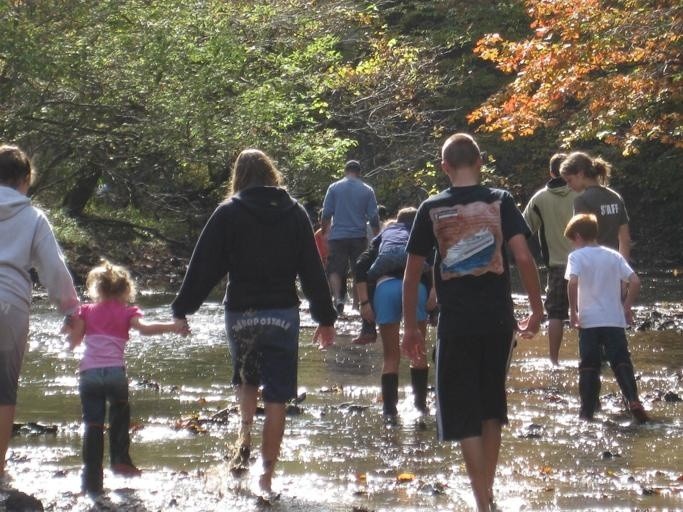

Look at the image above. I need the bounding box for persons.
[0,145,83,512]
[61,260,190,493]
[400,131,544,512]
[522,151,586,368]
[311,159,435,419]
[170,146,340,496]
[561,213,645,423]
[559,151,629,300]
